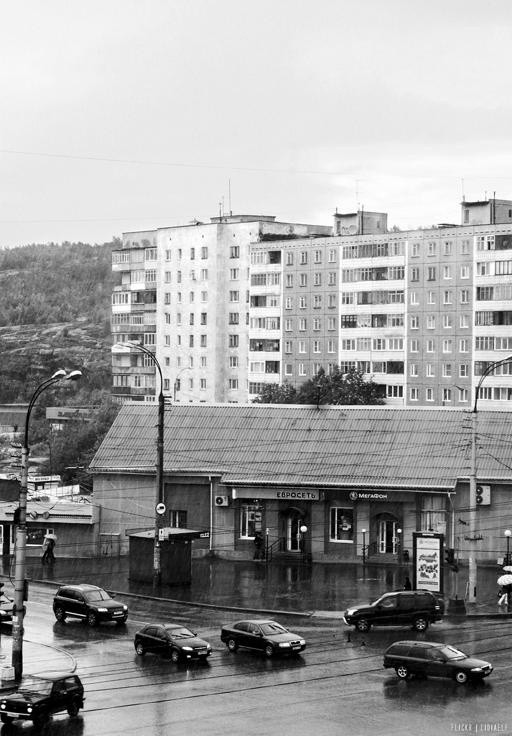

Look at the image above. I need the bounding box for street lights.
[10,367,85,680]
[115,340,165,598]
[361,528,367,578]
[503,529,512,566]
[300,525,308,579]
[396,528,403,564]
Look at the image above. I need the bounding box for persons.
[253,534,266,561]
[404,577,411,591]
[41,538,56,565]
[403,552,409,566]
[497,585,508,606]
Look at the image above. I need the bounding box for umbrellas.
[43,534,57,540]
[497,574,512,585]
[503,566,512,572]
[402,550,408,552]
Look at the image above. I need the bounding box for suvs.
[51,583,129,628]
[342,591,445,633]
[0,670,85,729]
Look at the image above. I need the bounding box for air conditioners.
[474,485,491,505]
[214,495,229,507]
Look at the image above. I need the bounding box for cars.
[133,622,214,664]
[382,640,494,684]
[219,619,307,657]
